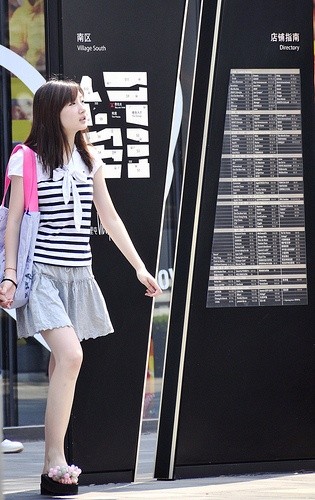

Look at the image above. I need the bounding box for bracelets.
[1,279,18,288]
[2,267,17,272]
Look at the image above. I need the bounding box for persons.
[1,76,160,495]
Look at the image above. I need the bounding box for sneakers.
[1,438,23,452]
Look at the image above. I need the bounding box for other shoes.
[40,465,82,495]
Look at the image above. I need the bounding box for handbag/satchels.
[0,143,40,308]
